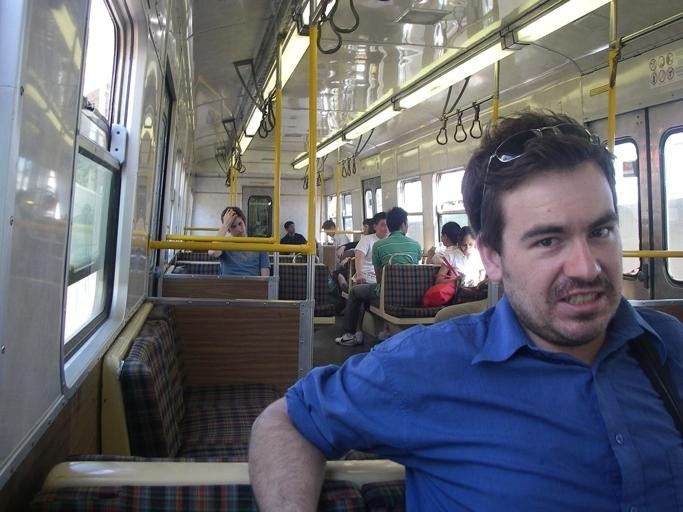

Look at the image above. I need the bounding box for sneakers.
[335,331,364,346]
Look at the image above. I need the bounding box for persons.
[352,212,392,346]
[279,220,308,244]
[426,221,463,266]
[322,221,351,247]
[208,207,272,278]
[328,218,378,312]
[334,207,422,347]
[436,226,487,304]
[248,110,683,509]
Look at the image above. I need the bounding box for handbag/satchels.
[423,277,458,306]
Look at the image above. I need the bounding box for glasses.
[479,124,596,230]
